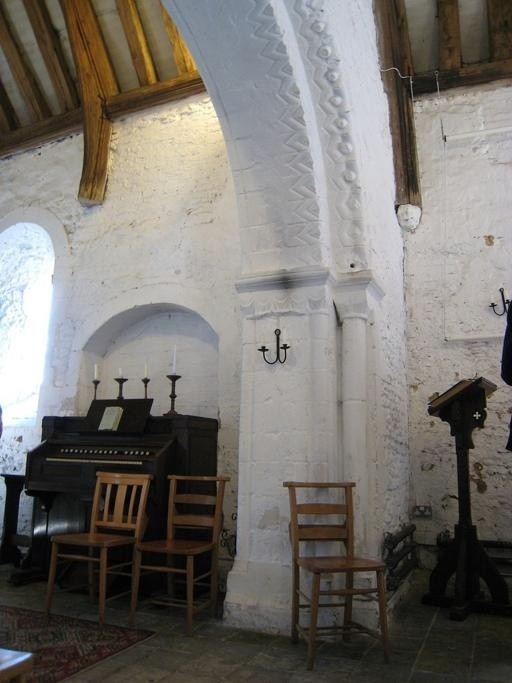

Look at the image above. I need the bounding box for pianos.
[7,398,216,597]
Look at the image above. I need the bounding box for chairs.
[44,472,390,671]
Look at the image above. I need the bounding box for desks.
[0,645,33,681]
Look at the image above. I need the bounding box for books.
[97,405,125,433]
[425,376,474,409]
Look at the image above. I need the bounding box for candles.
[94,344,178,381]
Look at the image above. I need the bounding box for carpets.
[0,604,156,683]
